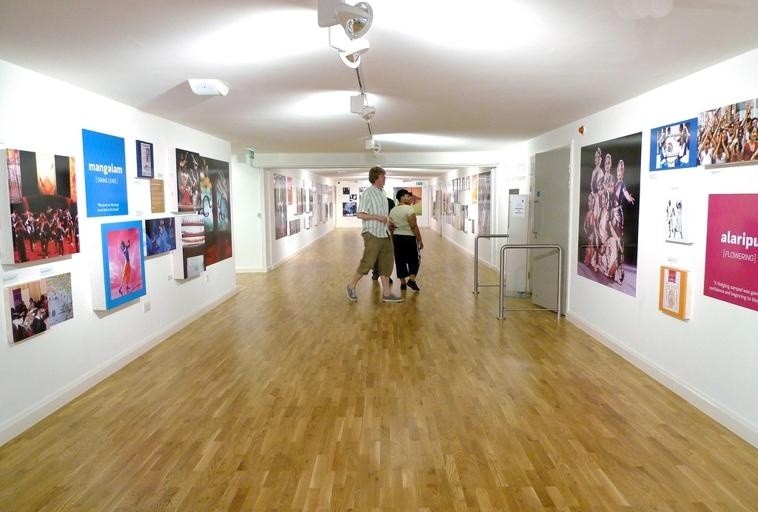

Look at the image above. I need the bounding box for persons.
[389,188,424,291]
[371,197,395,283]
[346,166,404,304]
[655,103,758,171]
[119,239,132,292]
[582,147,635,283]
[11,295,48,334]
[10,205,79,262]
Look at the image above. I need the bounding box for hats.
[396,189,412,200]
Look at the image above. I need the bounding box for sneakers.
[346,283,359,302]
[399,279,420,292]
[380,293,404,303]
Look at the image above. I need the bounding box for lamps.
[315,0,381,156]
